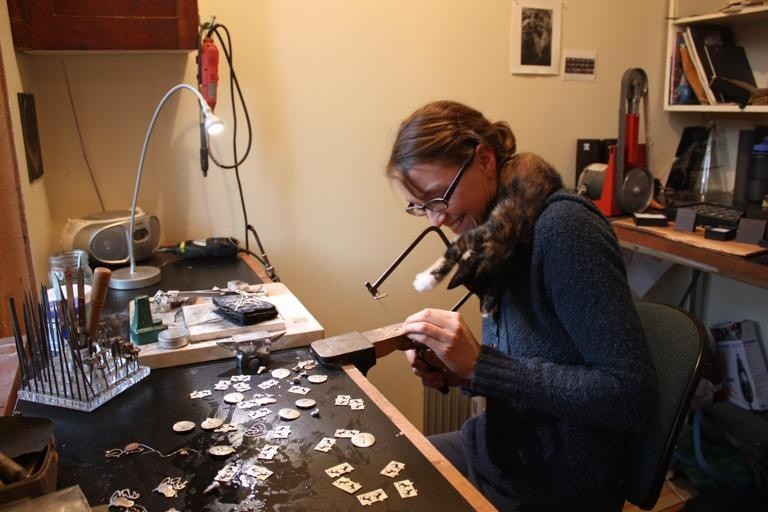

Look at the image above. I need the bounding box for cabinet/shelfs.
[6,0,200,57]
[663,0,768,114]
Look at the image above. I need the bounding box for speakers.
[576,140,602,192]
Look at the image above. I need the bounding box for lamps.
[108,84,226,290]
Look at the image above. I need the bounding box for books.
[667,25,758,105]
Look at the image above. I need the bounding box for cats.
[412,152,561,319]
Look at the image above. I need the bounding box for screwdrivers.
[86,268,112,385]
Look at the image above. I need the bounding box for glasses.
[405,154,476,216]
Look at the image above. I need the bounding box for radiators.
[421,385,473,436]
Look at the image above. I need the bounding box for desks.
[0,237,500,512]
[604,211,768,322]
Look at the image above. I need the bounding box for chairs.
[626,302,709,510]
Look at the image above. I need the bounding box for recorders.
[60,207,162,271]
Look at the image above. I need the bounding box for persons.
[383,99,658,511]
